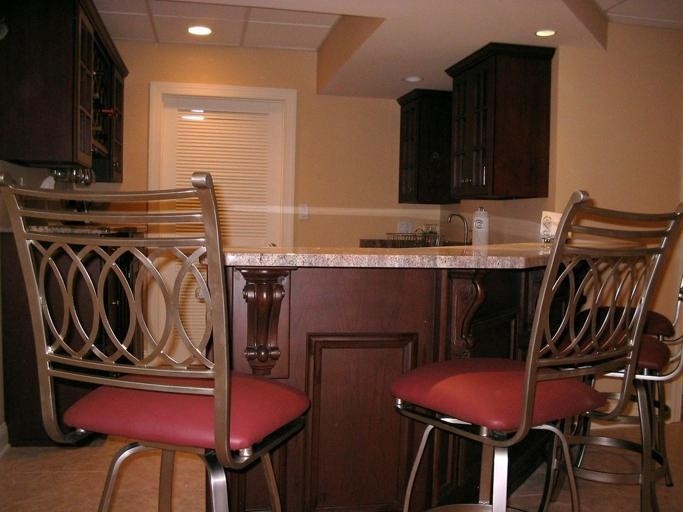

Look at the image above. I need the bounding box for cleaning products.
[473,207,489,246]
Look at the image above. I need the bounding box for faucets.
[448,213,468,245]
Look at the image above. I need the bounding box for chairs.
[1,172,311,512]
[550,276,683,512]
[392,188,683,511]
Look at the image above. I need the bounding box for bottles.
[471,206,490,246]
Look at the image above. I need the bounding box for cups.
[424,224,437,247]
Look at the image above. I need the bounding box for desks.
[147,242,649,512]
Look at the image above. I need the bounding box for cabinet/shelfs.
[444,41,556,200]
[398,88,464,204]
[0,1,130,447]
